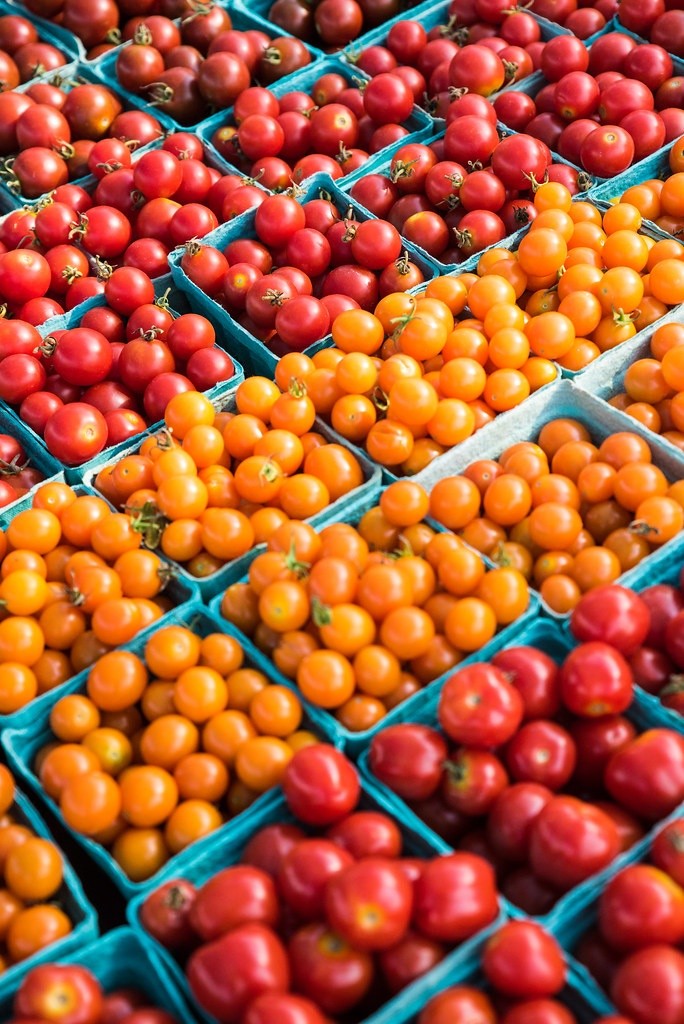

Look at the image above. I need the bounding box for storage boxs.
[0,0,684,1024]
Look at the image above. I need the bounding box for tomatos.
[0,0,684,1024]
